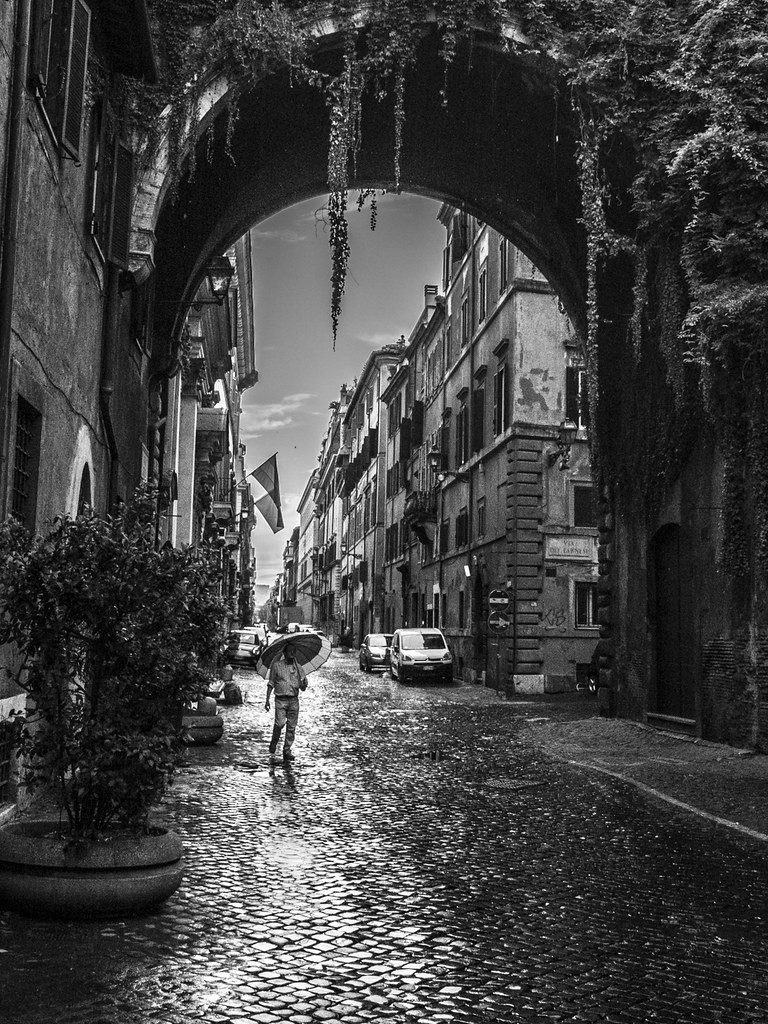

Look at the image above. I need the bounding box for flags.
[252,454,281,509]
[255,494,284,534]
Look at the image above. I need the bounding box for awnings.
[284,560,293,570]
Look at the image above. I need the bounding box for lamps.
[426,443,475,483]
[545,416,578,471]
[340,538,363,560]
[300,587,308,597]
[154,241,234,306]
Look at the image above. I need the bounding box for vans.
[386,627,455,685]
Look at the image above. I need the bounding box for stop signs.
[487,589,510,611]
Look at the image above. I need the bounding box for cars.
[285,622,325,638]
[359,633,395,672]
[243,622,273,651]
[217,629,265,671]
[587,642,609,694]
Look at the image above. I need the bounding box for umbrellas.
[256,631,332,679]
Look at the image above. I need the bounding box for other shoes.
[283,750,295,759]
[269,742,276,753]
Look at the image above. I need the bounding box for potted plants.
[0,473,233,915]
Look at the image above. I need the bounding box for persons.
[265,645,308,761]
[281,624,288,631]
